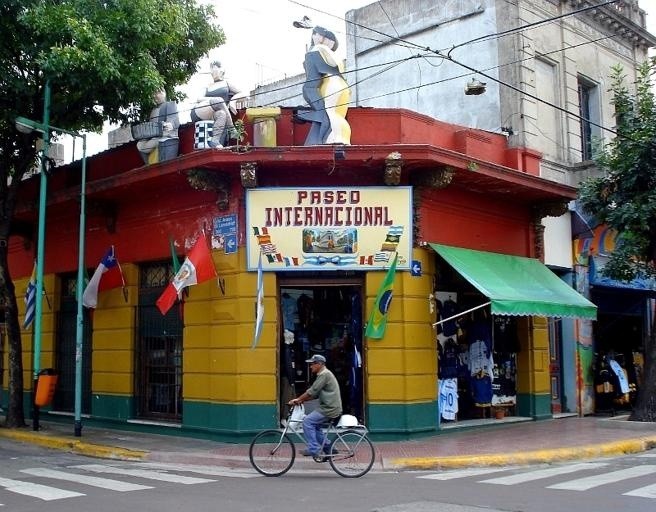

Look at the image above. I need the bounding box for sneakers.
[301,447,340,462]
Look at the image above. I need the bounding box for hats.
[304,353,327,363]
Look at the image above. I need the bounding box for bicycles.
[248,398,375,479]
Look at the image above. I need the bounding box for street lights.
[14,114,88,436]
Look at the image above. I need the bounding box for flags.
[154,231,219,317]
[249,252,270,347]
[363,253,399,340]
[20,259,46,332]
[79,245,127,310]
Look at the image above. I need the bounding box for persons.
[135,86,184,168]
[306,30,355,145]
[293,27,343,143]
[287,353,343,459]
[187,61,248,150]
